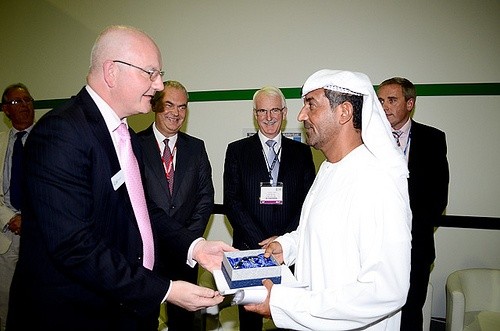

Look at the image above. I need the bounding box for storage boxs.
[221,248,281,289]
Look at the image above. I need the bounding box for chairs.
[446,268,500,331]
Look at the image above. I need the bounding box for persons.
[223,86,316,331]
[136,81,216,331]
[0,83,37,331]
[377,77,449,331]
[244,69,413,331]
[6,25,241,331]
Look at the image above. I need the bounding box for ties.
[116,124,155,271]
[161,139,175,196]
[392,132,403,146]
[266,140,280,187]
[9,131,27,212]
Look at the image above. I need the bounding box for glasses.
[254,107,285,115]
[6,97,35,105]
[113,60,166,82]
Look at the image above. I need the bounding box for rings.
[15,231,18,233]
